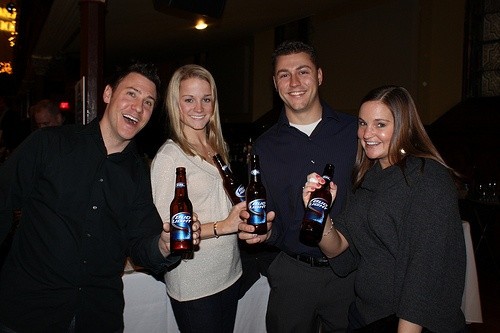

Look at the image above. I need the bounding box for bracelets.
[213,220,220,238]
[322,218,334,237]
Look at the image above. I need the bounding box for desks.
[468,197,500,265]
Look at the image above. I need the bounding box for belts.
[282,250,330,268]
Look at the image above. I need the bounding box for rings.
[302,186,305,191]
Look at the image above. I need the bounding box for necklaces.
[197,146,211,158]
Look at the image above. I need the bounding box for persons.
[0,63,201,333]
[238,40,359,333]
[302,83,467,333]
[29,98,63,128]
[149,64,247,333]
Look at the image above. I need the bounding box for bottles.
[247,136,253,154]
[298,163,335,247]
[213,153,246,205]
[170,167,194,259]
[247,153,267,235]
